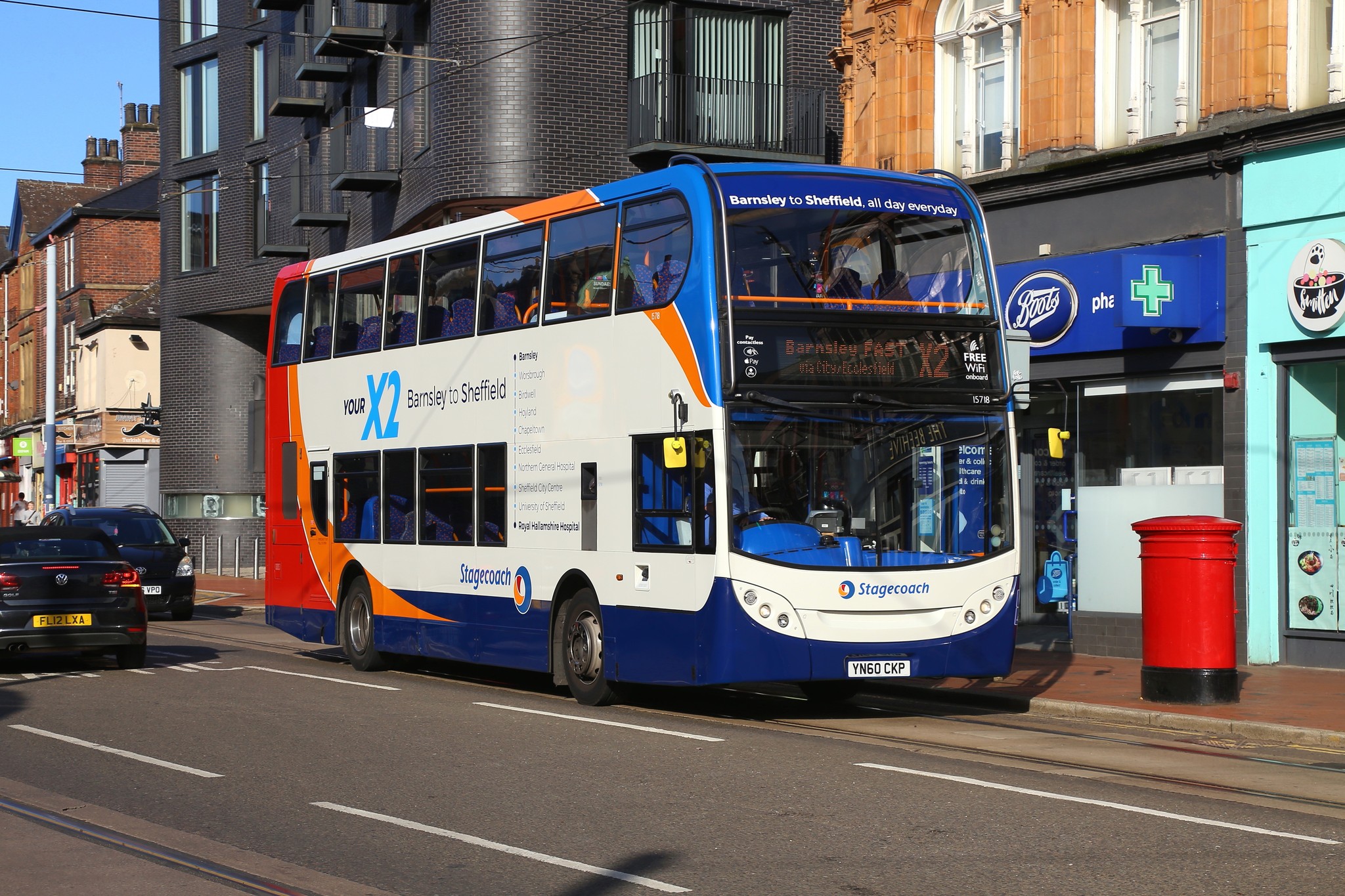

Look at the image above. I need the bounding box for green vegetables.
[1303,596,1322,613]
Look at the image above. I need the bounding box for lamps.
[84,338,98,352]
[128,334,144,343]
[67,344,84,352]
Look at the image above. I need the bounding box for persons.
[699,467,776,547]
[10,492,29,527]
[21,501,41,526]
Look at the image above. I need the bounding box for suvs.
[38,504,195,622]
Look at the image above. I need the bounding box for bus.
[264,153,1071,707]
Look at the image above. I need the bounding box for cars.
[0,524,147,664]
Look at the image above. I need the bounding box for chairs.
[651,258,686,305]
[357,315,382,351]
[463,519,504,543]
[632,263,655,307]
[870,268,924,313]
[373,493,414,539]
[310,325,333,357]
[720,257,755,306]
[115,524,148,543]
[446,297,476,336]
[425,304,454,340]
[399,509,446,541]
[339,319,365,354]
[339,496,360,539]
[489,291,522,330]
[423,521,459,542]
[278,343,301,363]
[387,309,416,346]
[824,266,873,312]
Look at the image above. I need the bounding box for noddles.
[1299,604,1318,615]
